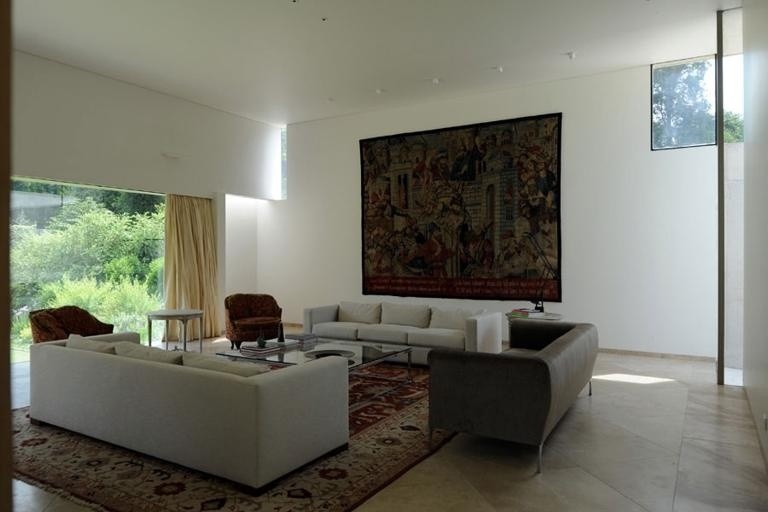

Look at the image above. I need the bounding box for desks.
[148,309,203,354]
[506,310,562,321]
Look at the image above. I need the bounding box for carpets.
[12,361,454,512]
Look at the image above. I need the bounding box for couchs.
[29,305,115,343]
[303,300,477,366]
[427,318,600,474]
[28,332,350,496]
[224,293,283,349]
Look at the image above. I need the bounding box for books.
[505,307,545,319]
[240,345,281,354]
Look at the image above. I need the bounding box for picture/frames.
[359,113,565,304]
